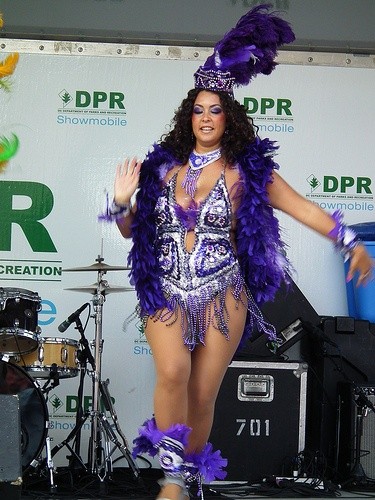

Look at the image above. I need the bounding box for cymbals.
[63,283,137,293]
[56,263,133,271]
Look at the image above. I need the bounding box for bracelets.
[328,209,361,263]
[96,188,132,222]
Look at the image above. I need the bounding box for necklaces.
[182,147,222,199]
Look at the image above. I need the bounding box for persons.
[97,4,375,500]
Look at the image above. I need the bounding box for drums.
[0,287,42,356]
[8,336,80,379]
[0,358,50,485]
